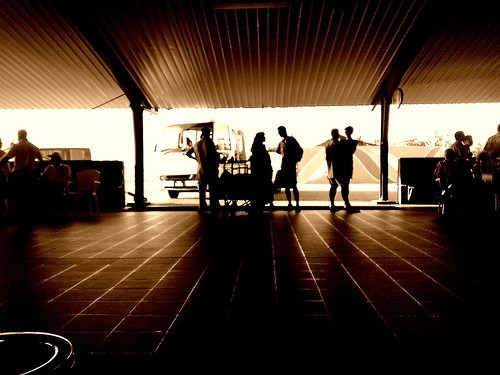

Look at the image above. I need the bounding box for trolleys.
[217,156,276,217]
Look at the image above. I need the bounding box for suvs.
[158,120,249,199]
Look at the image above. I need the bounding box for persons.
[187,127,219,210]
[249,132,277,217]
[437,122,500,229]
[0,128,72,228]
[324,128,361,214]
[270,126,302,212]
[339,126,356,200]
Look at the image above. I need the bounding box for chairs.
[76,169,100,217]
